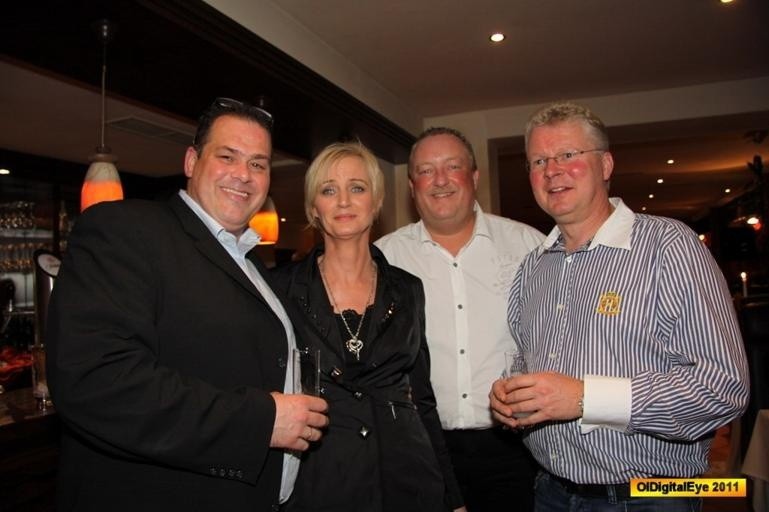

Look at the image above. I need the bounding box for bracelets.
[578,395,585,411]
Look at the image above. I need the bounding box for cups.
[292,345,321,398]
[30,345,50,400]
[503,349,536,430]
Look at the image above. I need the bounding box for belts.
[547,473,642,502]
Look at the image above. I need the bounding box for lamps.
[78,16,129,212]
[246,94,279,247]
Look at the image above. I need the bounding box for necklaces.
[319,259,377,361]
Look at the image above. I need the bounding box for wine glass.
[0,197,73,276]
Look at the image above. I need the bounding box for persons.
[267,138,469,511]
[486,100,753,510]
[374,126,549,512]
[45,99,331,511]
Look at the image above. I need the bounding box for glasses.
[210,96,277,131]
[525,147,608,174]
